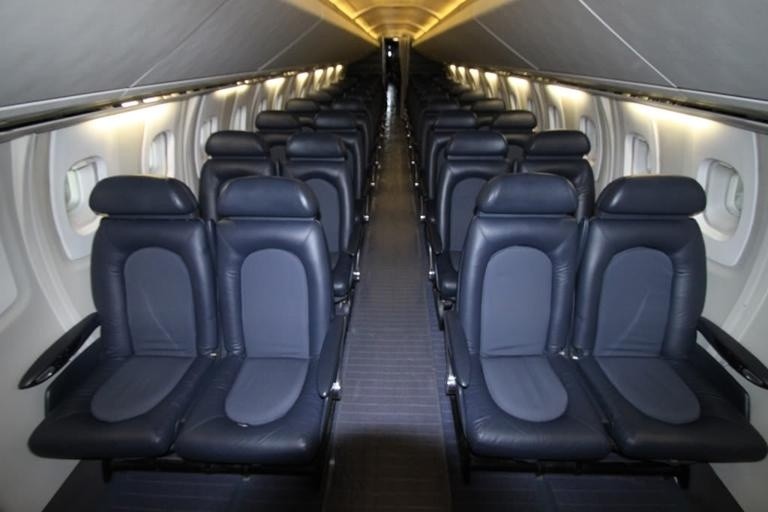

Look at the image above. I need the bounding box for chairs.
[426,129,523,296]
[282,136,362,304]
[470,97,509,116]
[171,174,344,476]
[18,174,208,475]
[313,110,362,138]
[335,98,367,112]
[442,169,613,476]
[421,96,468,116]
[286,97,319,115]
[195,130,278,218]
[419,115,476,134]
[514,130,597,216]
[487,108,539,136]
[253,109,304,152]
[578,172,764,477]
